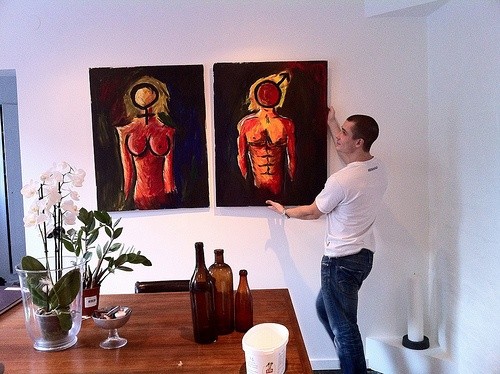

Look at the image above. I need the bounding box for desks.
[0,287,314,374]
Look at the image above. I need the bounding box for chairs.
[134,280,191,293]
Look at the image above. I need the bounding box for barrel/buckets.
[241,322,289,374]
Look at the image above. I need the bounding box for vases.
[14,255,87,351]
[34,304,72,342]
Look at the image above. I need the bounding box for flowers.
[21,159,87,331]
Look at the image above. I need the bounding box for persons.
[266,106,388,374]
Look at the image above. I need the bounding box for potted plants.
[46,206,152,317]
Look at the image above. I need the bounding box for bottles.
[234,269,254,333]
[208,249,234,336]
[189,241,218,344]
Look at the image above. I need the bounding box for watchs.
[283,208,290,219]
